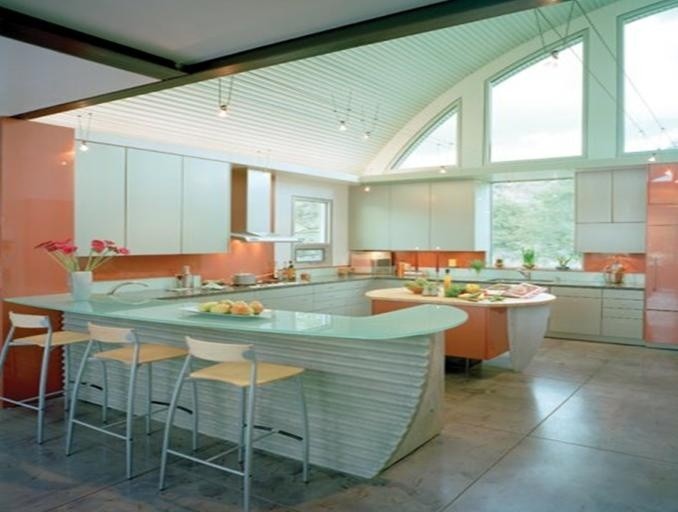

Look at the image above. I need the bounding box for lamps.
[217,76,234,116]
[77,114,92,151]
[331,94,351,132]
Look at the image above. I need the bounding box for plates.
[232,272,256,286]
[179,305,272,320]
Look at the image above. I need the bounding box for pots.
[602,261,626,286]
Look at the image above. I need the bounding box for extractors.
[229,233,299,244]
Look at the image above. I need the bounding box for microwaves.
[350,252,392,275]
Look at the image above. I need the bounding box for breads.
[249,300,262,311]
[203,300,216,311]
[229,304,252,317]
[219,297,235,309]
[210,303,229,313]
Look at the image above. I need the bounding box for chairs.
[0,311,108,446]
[157,336,310,512]
[64,322,197,481]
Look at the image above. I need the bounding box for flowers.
[32,237,129,271]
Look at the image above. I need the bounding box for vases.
[69,271,93,301]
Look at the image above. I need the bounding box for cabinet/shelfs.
[347,185,390,251]
[126,146,182,258]
[574,167,645,255]
[74,137,126,257]
[431,180,491,252]
[182,156,232,256]
[548,286,601,337]
[601,288,644,340]
[643,163,678,347]
[389,183,431,252]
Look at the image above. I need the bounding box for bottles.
[443,269,451,292]
